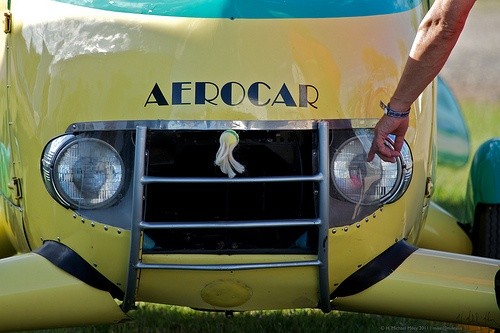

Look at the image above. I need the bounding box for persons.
[366,0,477,163]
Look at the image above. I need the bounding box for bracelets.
[379,100,411,118]
